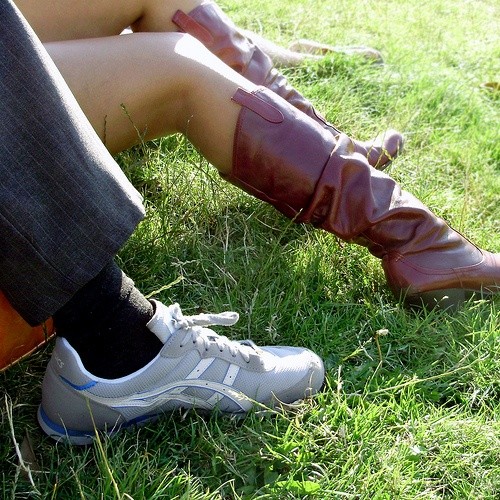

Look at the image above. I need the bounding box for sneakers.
[37,299,325,443]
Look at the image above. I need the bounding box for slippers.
[289,40,384,74]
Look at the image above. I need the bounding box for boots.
[218,86,500,309]
[173,1,404,178]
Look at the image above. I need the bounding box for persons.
[0,0,500,311]
[2,0,327,446]
